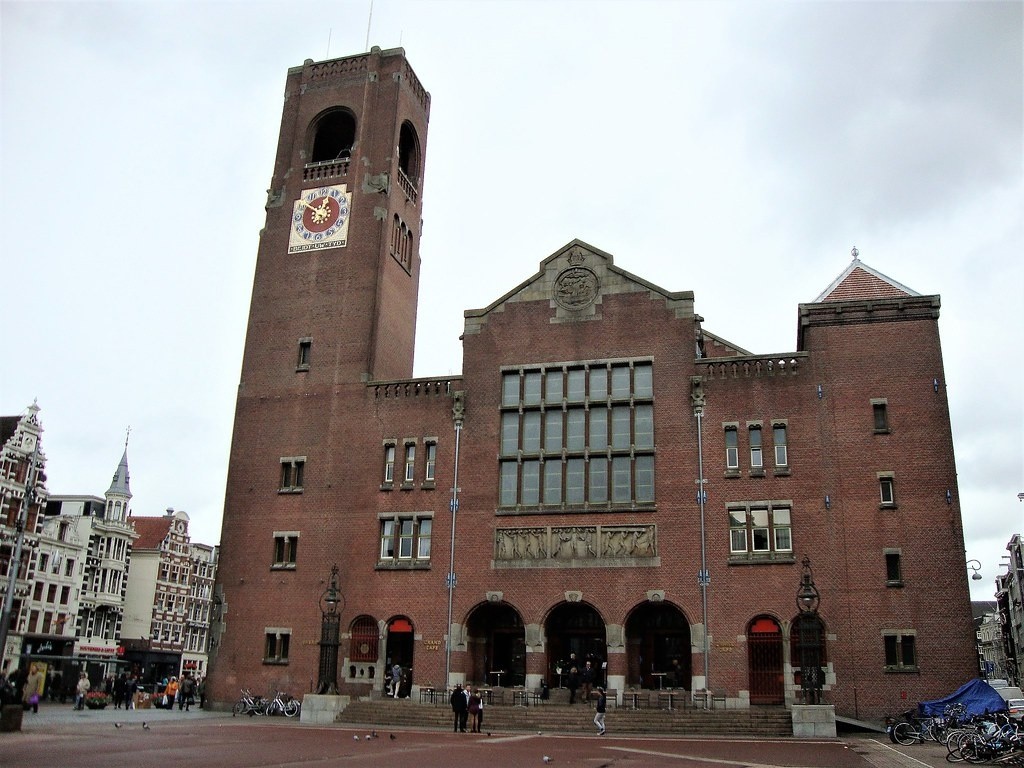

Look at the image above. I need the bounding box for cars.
[1006,699,1024,721]
[995,687,1023,701]
[983,680,1008,687]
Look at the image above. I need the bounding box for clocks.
[288,182,353,254]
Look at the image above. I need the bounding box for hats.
[597,687,604,691]
[171,676,175,679]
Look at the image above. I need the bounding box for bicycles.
[232,686,301,718]
[885,701,1024,767]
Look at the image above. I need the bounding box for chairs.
[529,688,544,707]
[637,694,650,709]
[674,694,687,710]
[589,691,600,708]
[493,691,505,705]
[606,689,618,709]
[713,694,726,710]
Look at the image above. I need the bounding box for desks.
[478,688,493,704]
[448,687,464,702]
[662,692,679,709]
[696,691,714,711]
[626,691,641,708]
[513,690,529,705]
[420,687,436,703]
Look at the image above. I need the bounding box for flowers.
[152,692,164,705]
[85,691,113,706]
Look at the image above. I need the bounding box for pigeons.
[114,722,124,729]
[372,729,380,739]
[364,734,372,740]
[389,733,398,741]
[352,733,360,741]
[141,720,151,731]
[537,730,542,735]
[486,732,491,737]
[541,753,555,765]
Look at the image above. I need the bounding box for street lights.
[791,555,826,704]
[312,563,341,695]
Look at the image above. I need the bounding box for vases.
[88,702,107,709]
[154,702,166,708]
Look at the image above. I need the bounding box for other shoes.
[394,696,399,699]
[388,693,393,695]
[597,730,605,735]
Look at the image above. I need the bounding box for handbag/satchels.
[163,696,168,705]
[188,697,195,705]
[130,702,135,709]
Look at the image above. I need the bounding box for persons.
[451,683,484,733]
[385,663,412,700]
[0,665,67,713]
[105,672,207,710]
[72,671,91,711]
[556,650,593,705]
[593,685,606,735]
